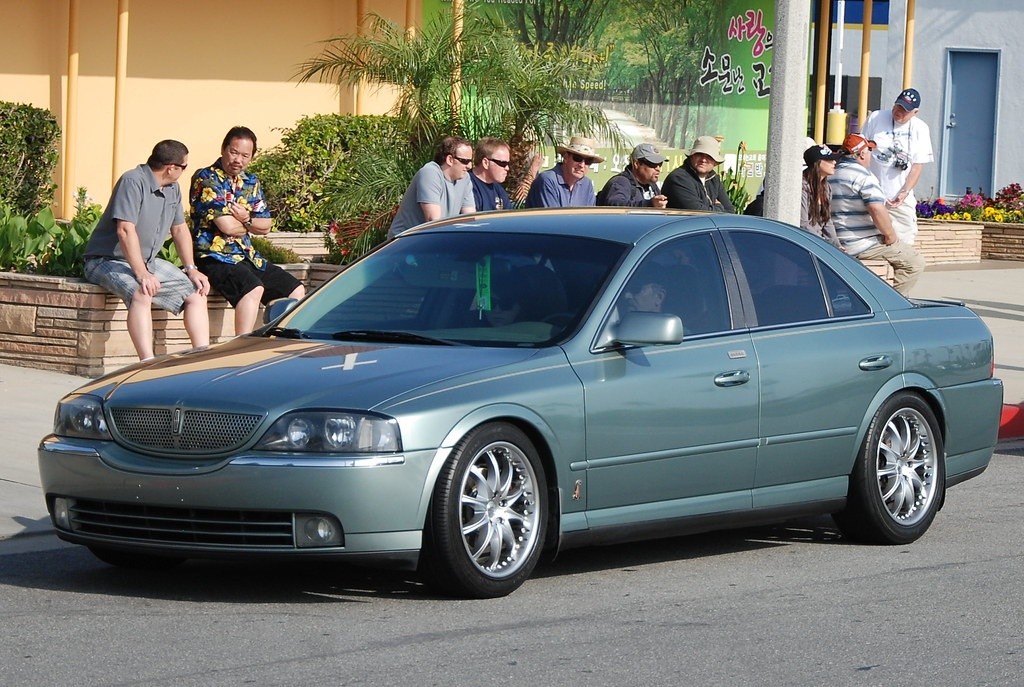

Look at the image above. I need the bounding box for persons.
[596,143,668,208]
[467,136,515,214]
[860,88,934,246]
[621,262,694,338]
[524,136,608,208]
[387,136,479,329]
[189,126,305,337]
[661,136,736,214]
[82,140,210,364]
[464,276,542,329]
[826,132,924,301]
[743,132,847,252]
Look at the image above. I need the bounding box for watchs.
[243,216,252,228]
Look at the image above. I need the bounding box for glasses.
[490,296,519,310]
[488,157,510,167]
[865,147,873,152]
[626,284,655,295]
[568,152,593,165]
[895,158,908,169]
[454,155,473,165]
[163,162,187,169]
[639,160,663,168]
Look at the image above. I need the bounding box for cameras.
[894,158,907,170]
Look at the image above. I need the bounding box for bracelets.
[900,189,909,195]
[184,265,198,272]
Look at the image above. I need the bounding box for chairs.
[458,258,541,325]
[669,264,711,334]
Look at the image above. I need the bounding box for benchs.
[754,285,828,327]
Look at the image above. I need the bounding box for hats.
[843,133,877,153]
[804,143,842,164]
[555,136,604,163]
[684,135,724,166]
[631,142,670,162]
[895,88,920,111]
[626,261,685,309]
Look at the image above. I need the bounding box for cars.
[38,207,1006,600]
